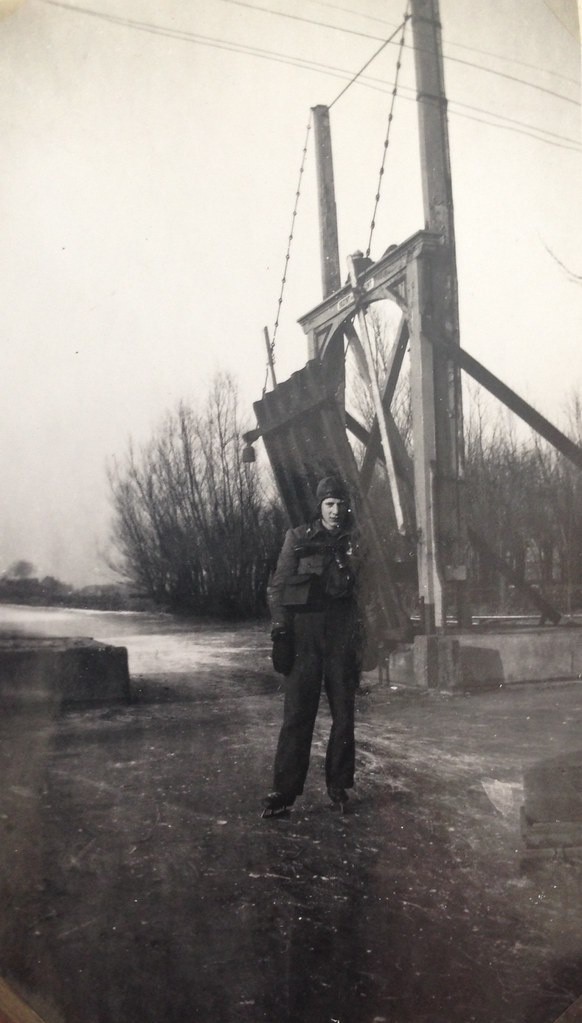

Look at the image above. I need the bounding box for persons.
[261,477,367,810]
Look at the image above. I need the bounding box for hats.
[314,478,350,508]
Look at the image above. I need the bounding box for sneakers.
[261,791,295,818]
[328,786,349,814]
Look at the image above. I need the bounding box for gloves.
[272,629,296,676]
